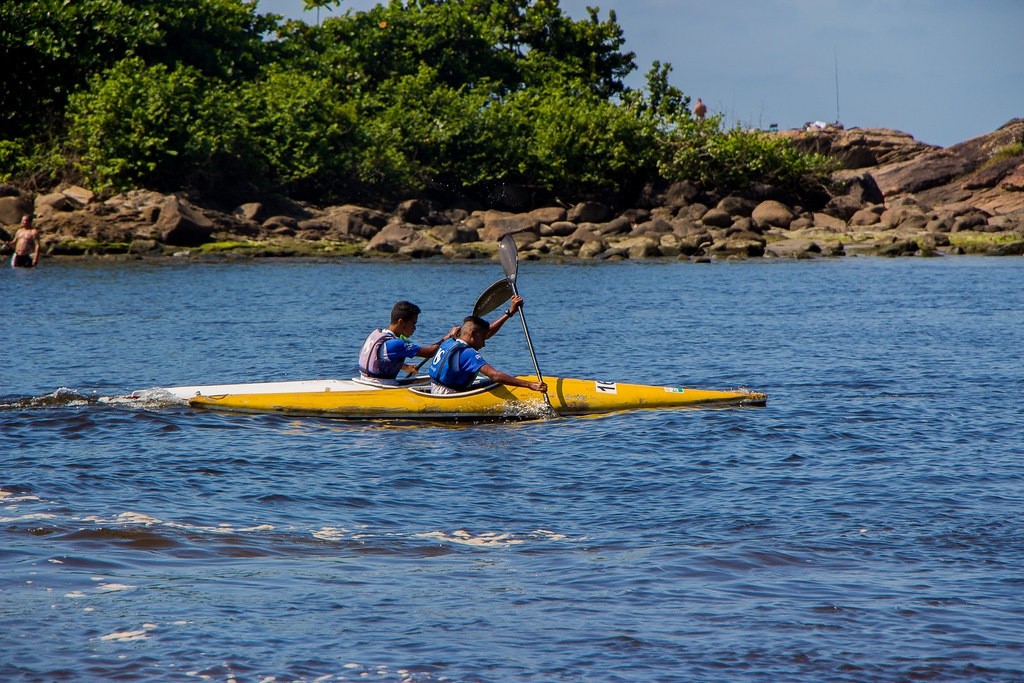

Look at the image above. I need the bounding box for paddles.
[405,276,514,378]
[499,234,551,406]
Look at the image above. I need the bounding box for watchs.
[505,308,513,318]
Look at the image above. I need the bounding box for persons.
[430,295,548,396]
[357,301,459,385]
[693,97,707,121]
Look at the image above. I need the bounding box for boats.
[50,371,769,418]
